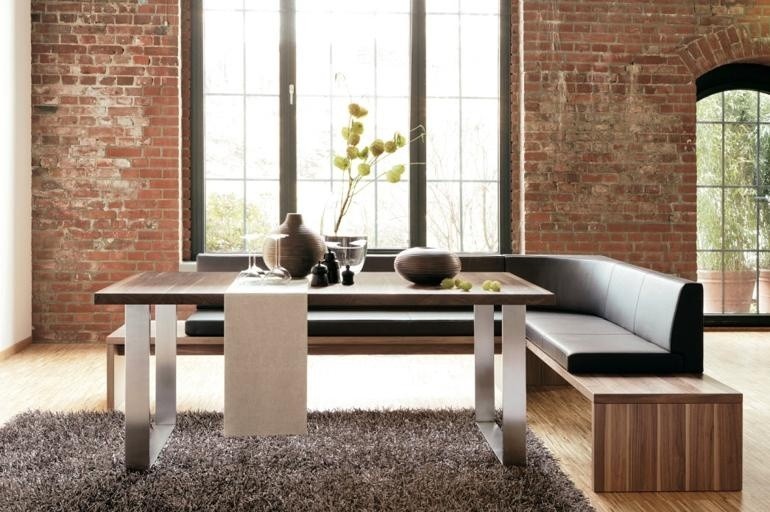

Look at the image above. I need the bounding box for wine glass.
[237,233,292,286]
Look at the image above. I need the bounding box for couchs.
[107,247,743,494]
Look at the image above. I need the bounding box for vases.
[320,232,373,278]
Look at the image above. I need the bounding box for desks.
[94,274,555,469]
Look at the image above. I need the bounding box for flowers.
[321,102,429,230]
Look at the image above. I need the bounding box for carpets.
[0,407,596,512]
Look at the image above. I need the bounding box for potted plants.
[694,88,770,315]
